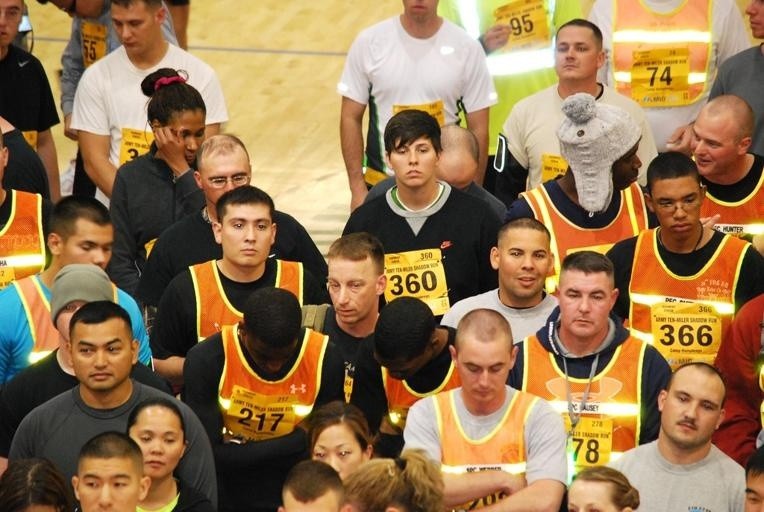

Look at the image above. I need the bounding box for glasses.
[208,176,247,188]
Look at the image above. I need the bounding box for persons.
[2,2,763,512]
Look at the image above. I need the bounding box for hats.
[51,264,113,328]
[558,92,642,217]
